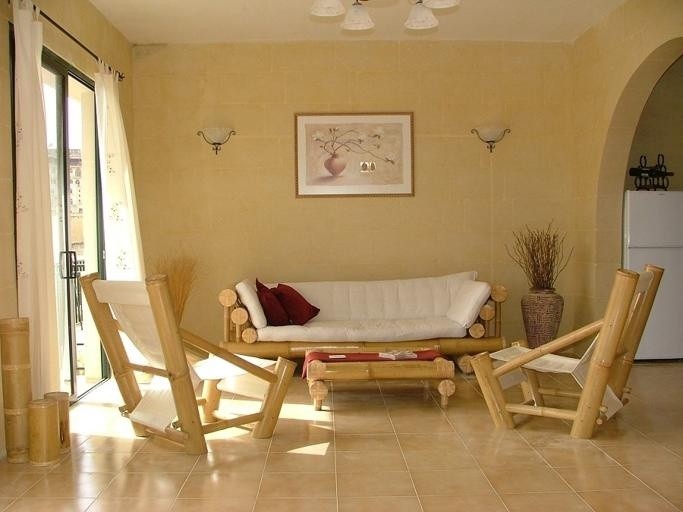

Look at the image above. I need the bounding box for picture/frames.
[295,112,414,198]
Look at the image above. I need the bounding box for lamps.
[309,0,460,30]
[470,127,511,152]
[196,127,236,155]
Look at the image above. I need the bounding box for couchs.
[217,270,507,375]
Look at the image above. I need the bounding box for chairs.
[79,270,299,455]
[470,264,665,439]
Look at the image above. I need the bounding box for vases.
[521,287,564,354]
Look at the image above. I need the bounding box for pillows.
[255,277,320,326]
